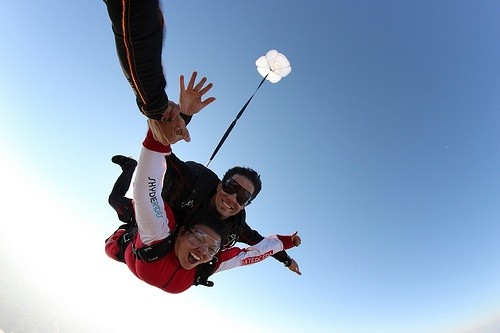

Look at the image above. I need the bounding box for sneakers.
[112,155,138,170]
[118,224,127,229]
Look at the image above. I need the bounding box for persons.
[102,0,191,146]
[108,71,302,275]
[105,101,302,295]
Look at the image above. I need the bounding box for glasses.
[222,176,252,207]
[186,229,221,258]
